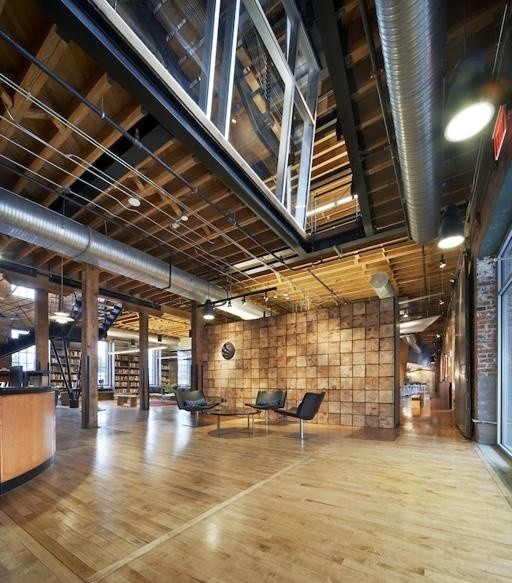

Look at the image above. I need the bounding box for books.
[50,348,82,390]
[114,353,140,394]
[161,363,169,387]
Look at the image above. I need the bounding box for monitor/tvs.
[8,365,23,386]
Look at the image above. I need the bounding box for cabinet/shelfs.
[115,351,140,395]
[161,359,170,387]
[50,339,84,394]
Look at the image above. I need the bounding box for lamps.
[436,201,466,249]
[439,253,446,268]
[203,299,215,320]
[444,7,492,143]
[47,303,74,323]
[197,286,277,308]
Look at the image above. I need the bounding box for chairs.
[176,388,325,439]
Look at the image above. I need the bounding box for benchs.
[114,393,140,406]
